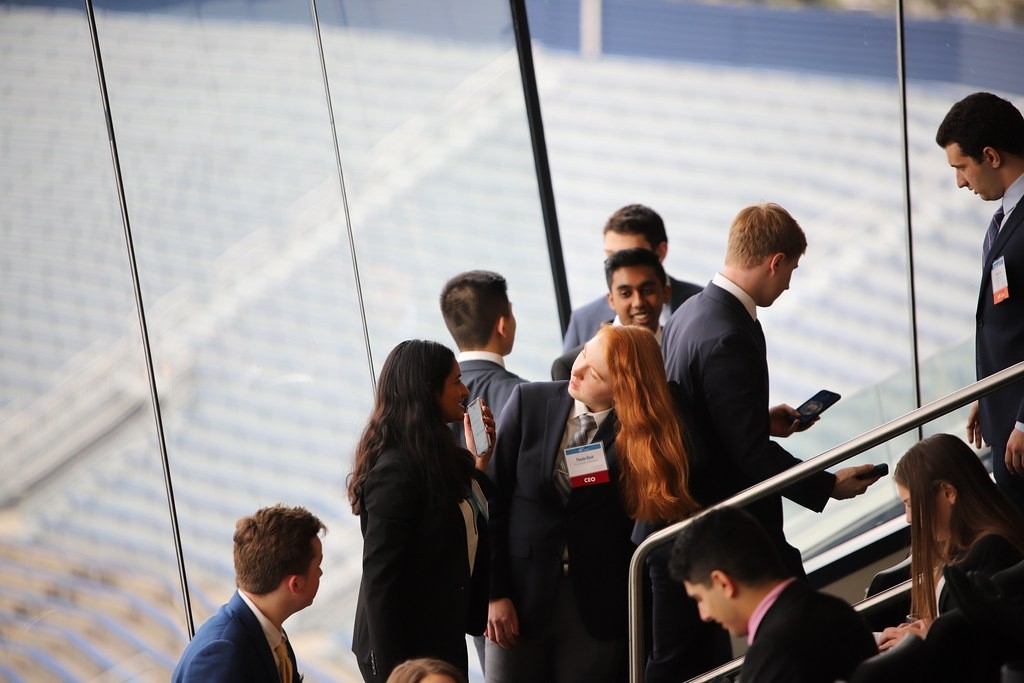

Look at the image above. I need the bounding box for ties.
[981,205,1004,269]
[276,636,293,683]
[553,414,597,506]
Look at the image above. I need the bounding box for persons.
[935,93,1024,496]
[176,508,325,683]
[347,342,498,683]
[660,201,881,573]
[484,325,698,682]
[440,271,536,674]
[876,433,1023,683]
[560,205,706,352]
[386,658,467,683]
[552,248,668,378]
[665,504,880,683]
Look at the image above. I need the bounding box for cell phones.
[856,463,889,480]
[464,396,491,457]
[788,390,841,426]
